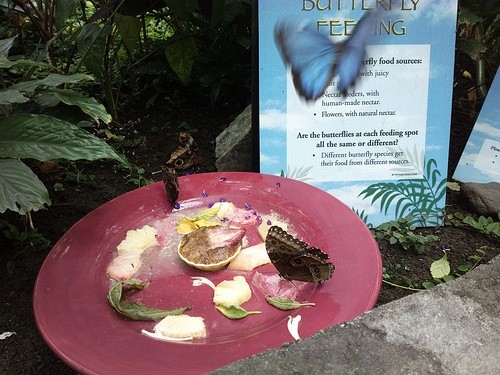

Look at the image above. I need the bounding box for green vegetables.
[107,278,315,322]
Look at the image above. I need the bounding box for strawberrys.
[217,228,247,246]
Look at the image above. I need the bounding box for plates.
[178,232,241,273]
[32,171,384,375]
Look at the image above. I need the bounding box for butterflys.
[274,0,404,102]
[265,225,335,283]
[159,162,179,203]
